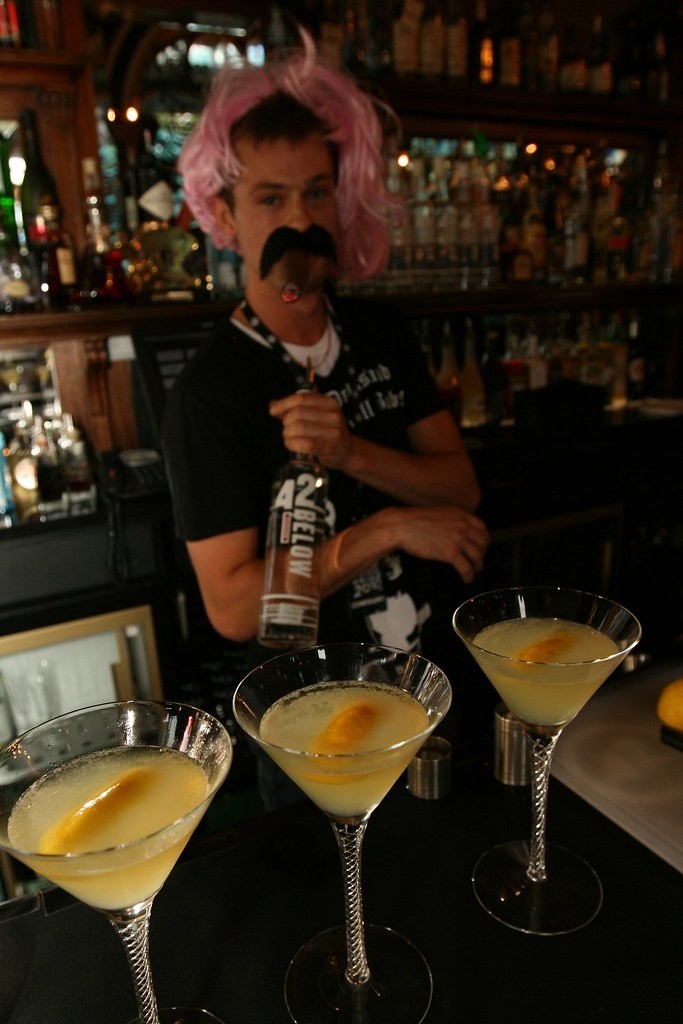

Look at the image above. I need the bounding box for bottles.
[417,310,649,427]
[258,382,331,652]
[0,109,245,315]
[265,5,289,58]
[0,361,96,528]
[318,1,670,101]
[338,136,678,296]
[0,0,21,47]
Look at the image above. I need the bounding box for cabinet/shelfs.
[0,0,683,454]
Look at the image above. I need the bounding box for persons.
[155,56,489,695]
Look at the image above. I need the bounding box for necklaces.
[293,316,334,365]
[239,297,357,395]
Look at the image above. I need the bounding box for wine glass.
[0,699,232,1024]
[452,584,642,937]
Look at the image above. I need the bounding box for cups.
[409,736,452,800]
[493,700,531,787]
[232,642,452,1024]
